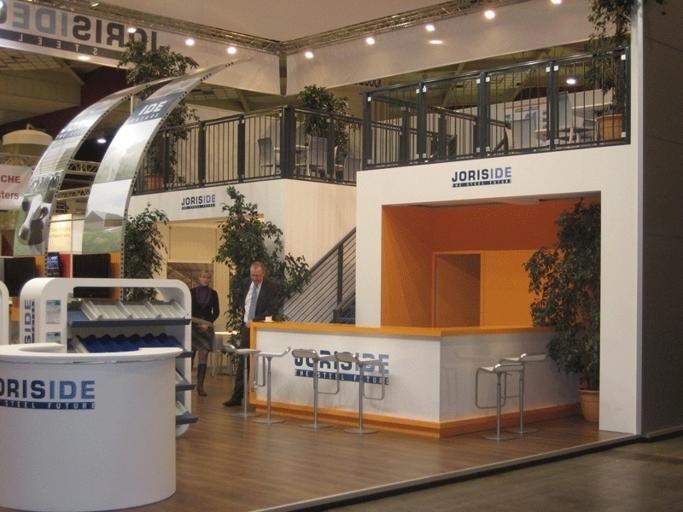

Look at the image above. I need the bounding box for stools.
[476,351,546,441]
[221,344,385,435]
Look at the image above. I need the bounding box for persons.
[222,260,279,406]
[191,270,218,397]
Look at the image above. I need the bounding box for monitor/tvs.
[48,253,59,269]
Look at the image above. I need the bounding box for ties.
[248,287,258,321]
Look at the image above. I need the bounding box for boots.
[195,364,208,397]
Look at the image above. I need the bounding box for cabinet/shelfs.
[20,277,197,437]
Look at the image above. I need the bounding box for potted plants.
[581,0,636,142]
[116,37,215,192]
[521,198,600,423]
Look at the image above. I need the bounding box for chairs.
[208,332,236,376]
[257,137,361,185]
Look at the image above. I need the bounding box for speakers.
[0,257,36,297]
[73,253,111,298]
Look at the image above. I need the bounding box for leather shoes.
[223,398,242,407]
